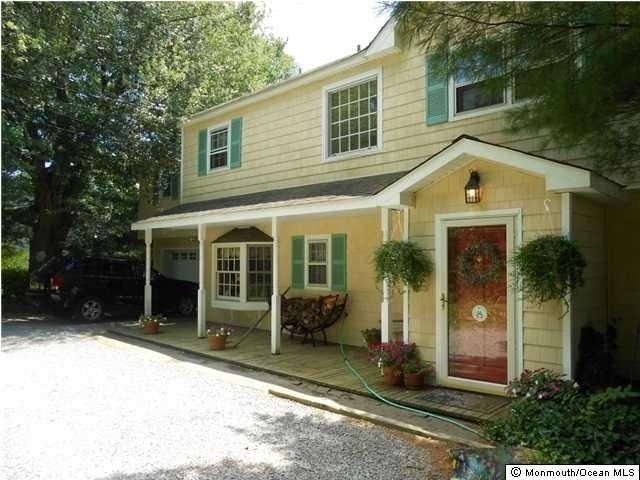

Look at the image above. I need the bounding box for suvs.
[29,256,198,325]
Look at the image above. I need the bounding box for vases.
[144,320,160,335]
[405,372,425,390]
[206,335,227,351]
[383,364,403,386]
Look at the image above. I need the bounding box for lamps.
[463,170,484,205]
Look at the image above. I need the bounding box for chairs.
[265,285,349,349]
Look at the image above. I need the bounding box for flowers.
[139,313,167,327]
[207,327,233,338]
[396,359,436,375]
[367,341,418,378]
